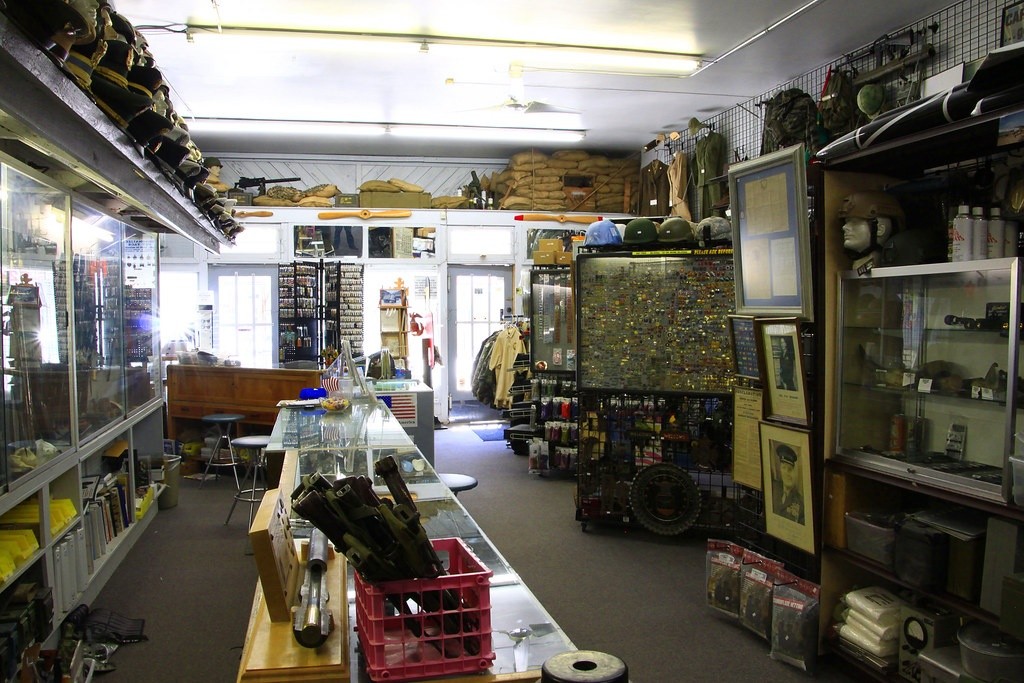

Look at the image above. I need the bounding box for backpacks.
[822,74,853,130]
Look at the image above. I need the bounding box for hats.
[837,190,904,221]
[0,0,245,244]
[687,117,710,138]
[776,444,797,469]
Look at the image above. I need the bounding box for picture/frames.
[758,421,817,558]
[727,315,763,382]
[753,317,811,429]
[727,142,815,322]
[732,385,763,491]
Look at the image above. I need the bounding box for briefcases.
[359,191,433,211]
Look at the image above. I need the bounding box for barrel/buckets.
[158,454,182,509]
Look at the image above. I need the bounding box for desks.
[236,446,579,683]
[166,363,348,466]
[265,400,414,489]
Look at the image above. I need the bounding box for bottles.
[988,208,1004,258]
[972,208,988,260]
[1005,221,1016,256]
[952,205,974,262]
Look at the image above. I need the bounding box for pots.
[958,622,1024,683]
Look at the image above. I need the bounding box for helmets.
[623,217,731,245]
[857,83,886,119]
[205,157,223,170]
[584,220,622,247]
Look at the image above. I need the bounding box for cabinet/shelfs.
[0,15,234,683]
[379,288,409,370]
[817,100,1024,683]
[508,353,539,442]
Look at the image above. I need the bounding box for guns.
[234,176,302,196]
[835,29,914,70]
[290,455,481,660]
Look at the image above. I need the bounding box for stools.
[438,472,478,497]
[199,414,245,491]
[224,436,271,535]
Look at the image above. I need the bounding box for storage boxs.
[844,513,897,565]
[895,521,948,589]
[1009,456,1024,507]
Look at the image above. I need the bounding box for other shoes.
[711,195,729,208]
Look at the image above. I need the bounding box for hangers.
[496,315,530,333]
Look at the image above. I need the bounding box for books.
[52,472,132,613]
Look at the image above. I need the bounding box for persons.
[202,157,226,192]
[666,131,728,220]
[776,337,797,392]
[769,445,806,525]
[839,191,905,271]
